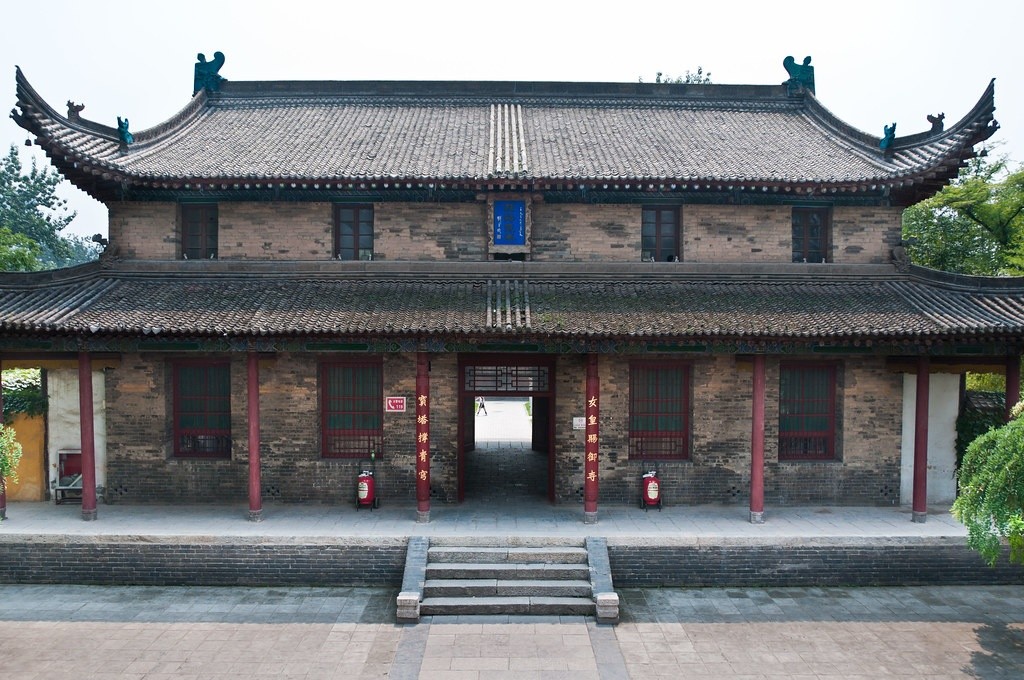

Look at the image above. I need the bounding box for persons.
[476,396,487,416]
